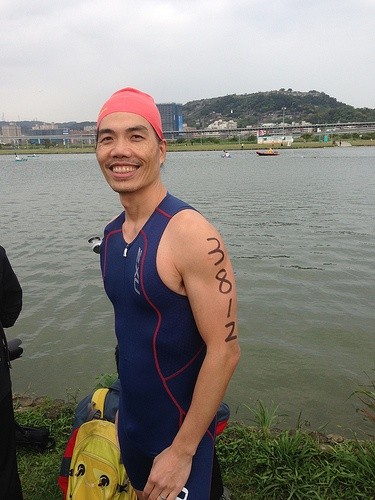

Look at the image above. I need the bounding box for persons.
[95,87,241,500]
[0,246,23,500]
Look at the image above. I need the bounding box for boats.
[27,155,40,157]
[220,150,232,159]
[15,159,28,162]
[256,147,280,156]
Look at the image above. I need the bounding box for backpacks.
[64,387,139,500]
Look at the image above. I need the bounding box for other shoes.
[18,425,49,446]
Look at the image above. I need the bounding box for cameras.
[5,338,23,362]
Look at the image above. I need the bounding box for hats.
[95,87,163,141]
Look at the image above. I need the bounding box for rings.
[160,494,166,500]
